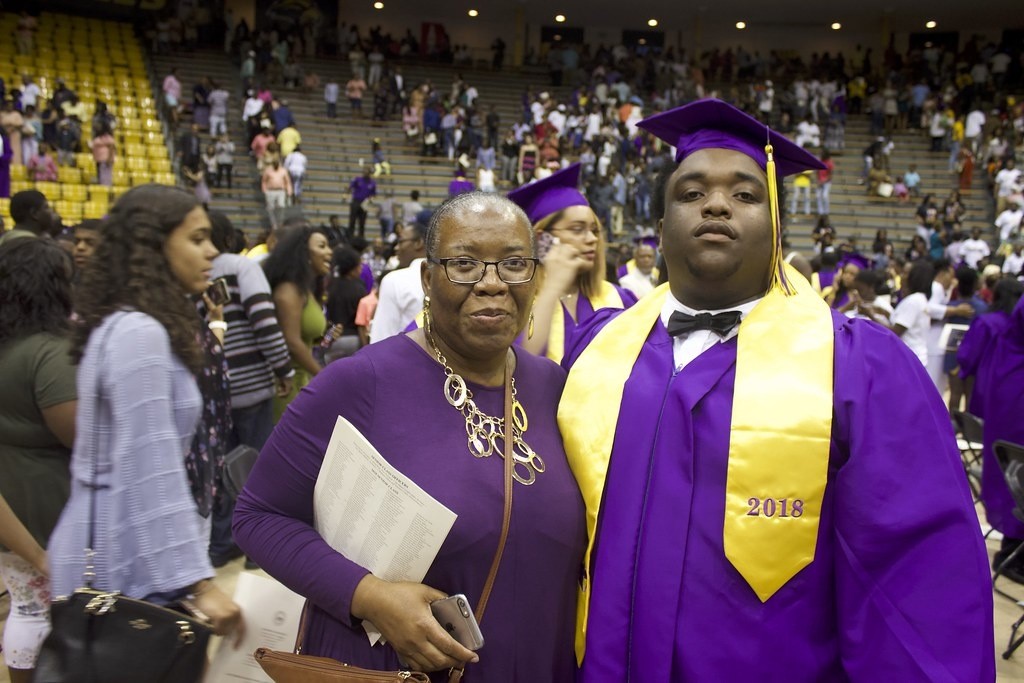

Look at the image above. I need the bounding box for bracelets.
[194,583,212,596]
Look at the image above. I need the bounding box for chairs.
[0,0,182,232]
[989,439,1024,660]
[955,412,985,504]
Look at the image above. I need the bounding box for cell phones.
[396,594,485,668]
[206,278,230,306]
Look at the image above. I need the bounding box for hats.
[635,97,829,190]
[632,235,660,248]
[509,162,588,224]
[840,250,875,270]
[335,246,361,274]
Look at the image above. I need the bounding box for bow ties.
[664,306,744,338]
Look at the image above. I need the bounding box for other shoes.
[210,547,243,568]
[245,556,260,569]
[992,551,1024,584]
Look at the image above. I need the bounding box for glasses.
[423,249,542,284]
[396,234,418,246]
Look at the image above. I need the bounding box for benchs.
[152,38,1024,264]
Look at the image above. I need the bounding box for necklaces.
[559,288,579,300]
[429,334,545,486]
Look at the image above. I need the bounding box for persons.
[0,190,102,683]
[185,210,426,570]
[150,0,500,198]
[48,183,245,683]
[557,97,995,683]
[233,191,589,683]
[500,35,1024,222]
[162,65,308,229]
[0,72,116,197]
[503,163,668,374]
[344,138,425,241]
[782,214,1024,683]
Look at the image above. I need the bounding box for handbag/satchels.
[50,584,213,683]
[250,644,445,683]
